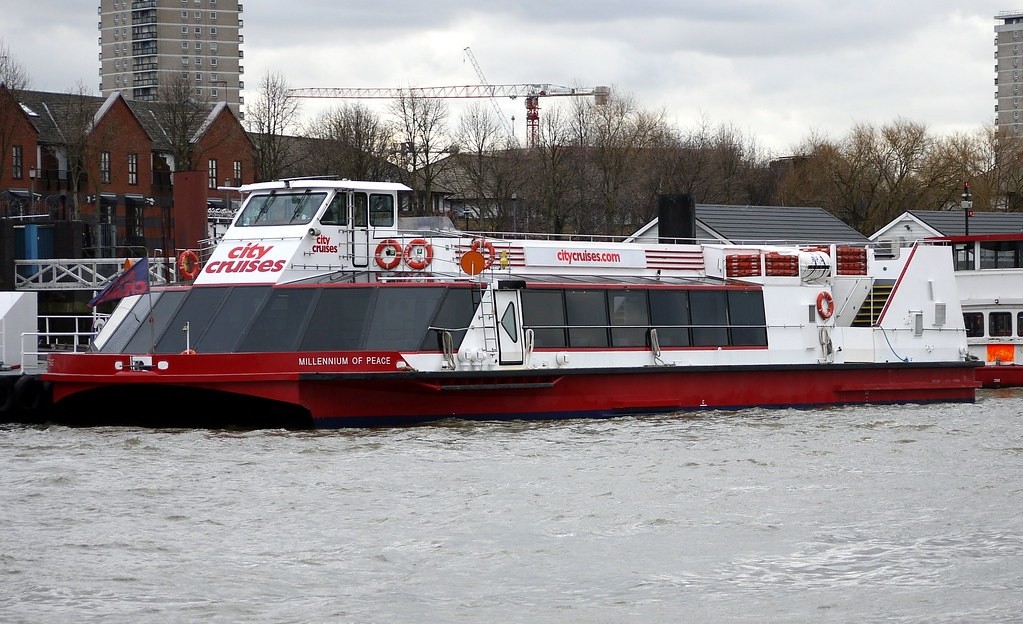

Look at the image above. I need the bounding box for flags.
[85,255,150,308]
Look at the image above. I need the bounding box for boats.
[13,172,986,422]
[924,232,1023,390]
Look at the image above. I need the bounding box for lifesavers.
[471,240,495,270]
[180,348,197,355]
[817,291,835,320]
[178,250,200,281]
[405,240,433,270]
[375,239,403,271]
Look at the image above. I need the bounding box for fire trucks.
[277,82,614,177]
